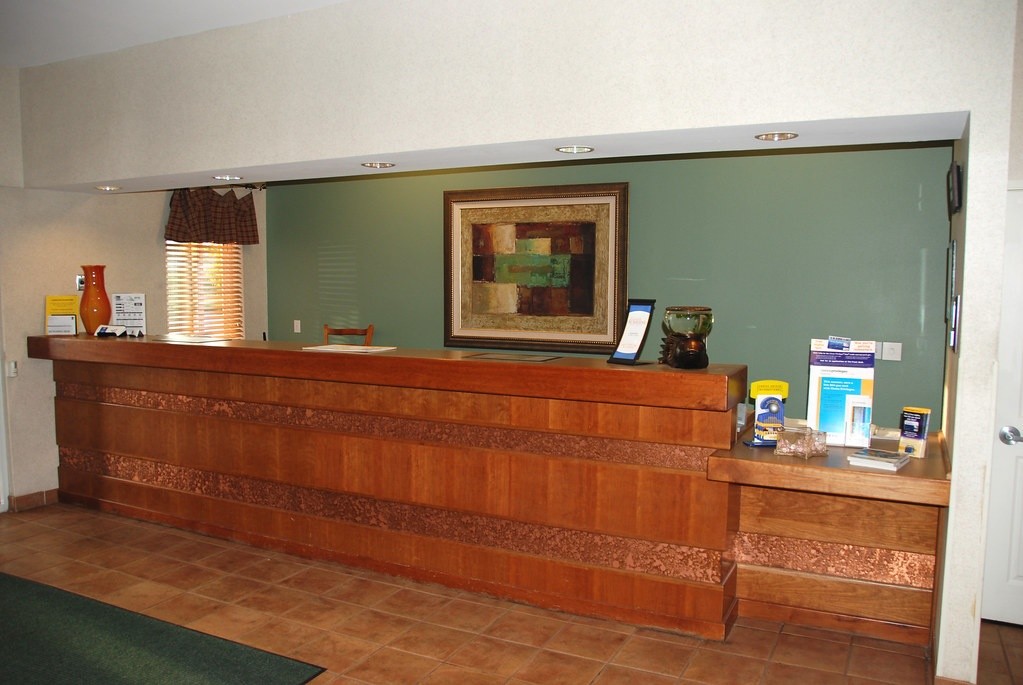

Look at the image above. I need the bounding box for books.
[846,447,911,472]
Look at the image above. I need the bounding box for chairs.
[322,324,375,346]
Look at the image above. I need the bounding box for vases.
[79,264,111,334]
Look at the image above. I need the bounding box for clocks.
[947,161,960,218]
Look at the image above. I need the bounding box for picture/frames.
[443,182,628,355]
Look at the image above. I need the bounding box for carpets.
[0,570,328,685]
